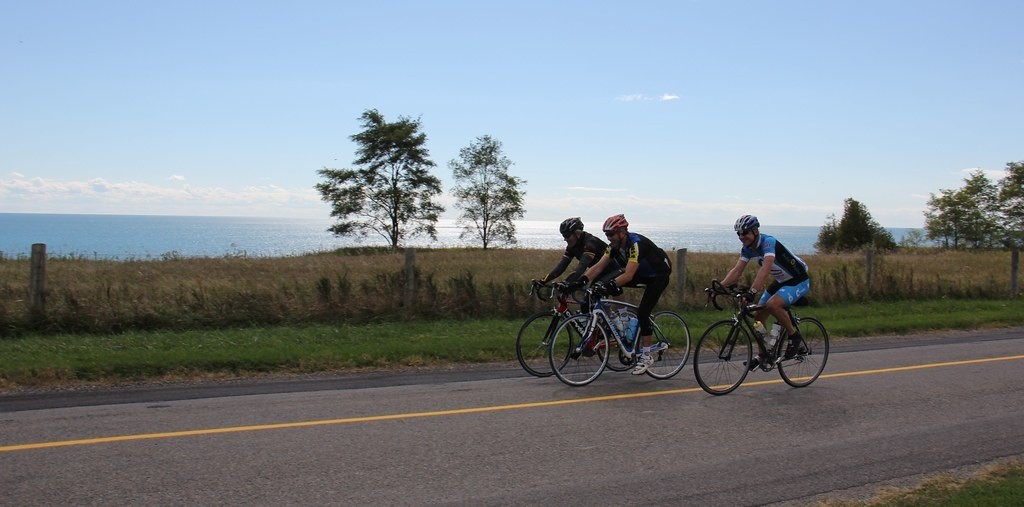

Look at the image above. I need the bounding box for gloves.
[534,279,545,290]
[555,281,566,293]
[564,275,589,295]
[598,280,618,296]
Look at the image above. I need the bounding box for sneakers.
[632,355,654,375]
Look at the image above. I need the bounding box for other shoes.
[785,332,802,360]
[743,357,759,372]
[599,323,611,340]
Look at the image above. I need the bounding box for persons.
[707,214,811,373]
[570,213,672,375]
[532,218,631,341]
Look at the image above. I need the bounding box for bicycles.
[516,278,644,378]
[549,279,691,386]
[693,279,830,397]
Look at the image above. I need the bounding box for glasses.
[605,231,618,237]
[737,228,754,236]
[564,231,574,239]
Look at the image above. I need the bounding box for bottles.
[626,318,638,346]
[753,321,769,342]
[610,311,626,337]
[769,321,784,346]
[574,310,587,327]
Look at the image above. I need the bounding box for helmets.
[734,215,760,231]
[603,214,628,232]
[560,218,584,237]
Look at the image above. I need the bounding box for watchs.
[749,288,757,294]
